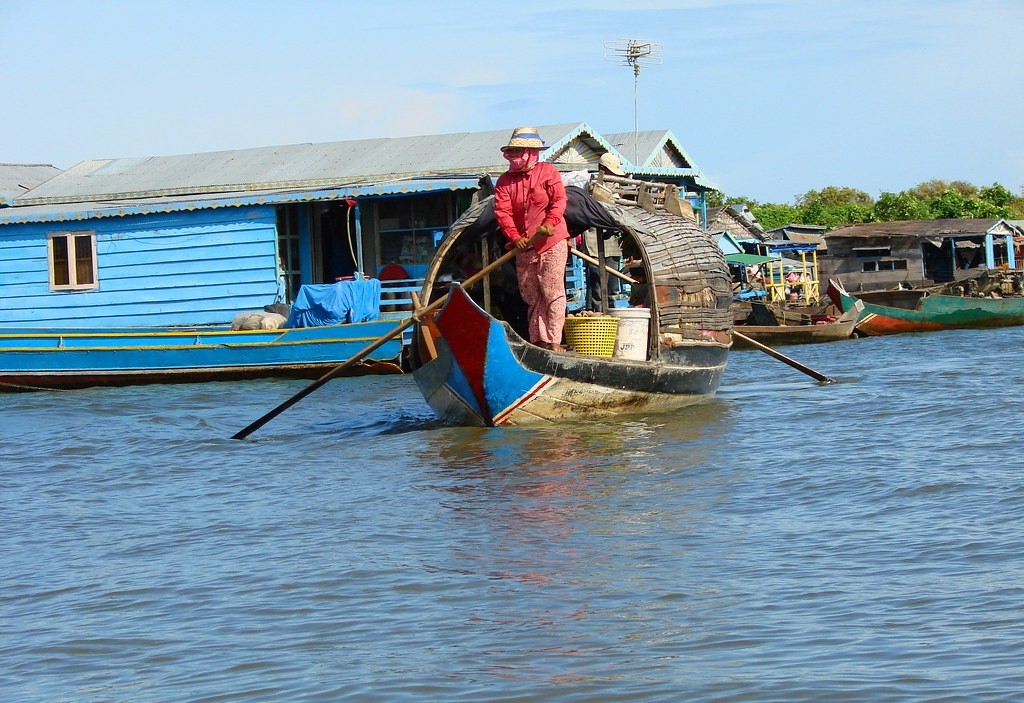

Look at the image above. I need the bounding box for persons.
[581,153,634,312]
[494,127,570,353]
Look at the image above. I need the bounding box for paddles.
[230,223,546,441]
[566,240,828,381]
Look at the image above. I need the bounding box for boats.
[826,278,1024,335]
[410,178,734,426]
[732,298,864,347]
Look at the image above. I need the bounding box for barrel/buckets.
[606,306,651,361]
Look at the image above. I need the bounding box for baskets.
[563,316,621,358]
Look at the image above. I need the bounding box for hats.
[600,153,626,175]
[500,126,551,152]
